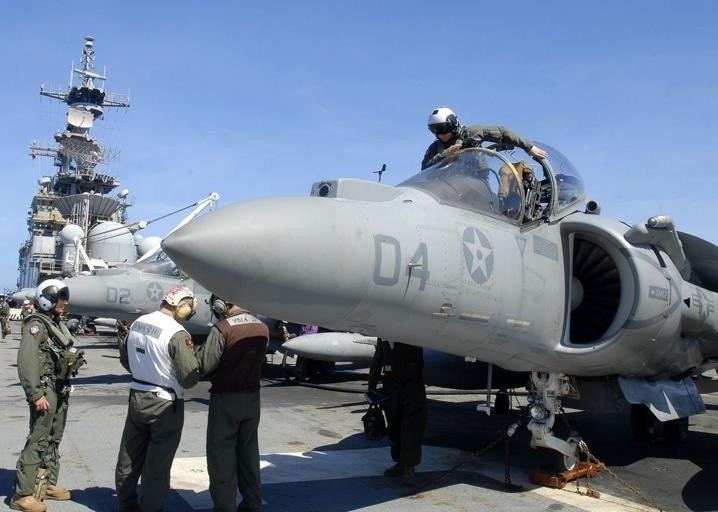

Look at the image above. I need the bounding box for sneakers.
[10,494,48,512]
[384,464,416,487]
[46,483,71,501]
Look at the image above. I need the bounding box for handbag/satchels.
[362,408,387,441]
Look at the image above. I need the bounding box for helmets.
[33,279,69,312]
[162,285,199,321]
[209,293,234,320]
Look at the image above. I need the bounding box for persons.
[116,319,131,355]
[366,337,429,488]
[194,293,269,512]
[294,324,318,385]
[1,294,35,342]
[114,285,199,511]
[7,277,86,512]
[420,107,548,182]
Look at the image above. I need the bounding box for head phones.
[211,294,228,315]
[175,301,191,318]
